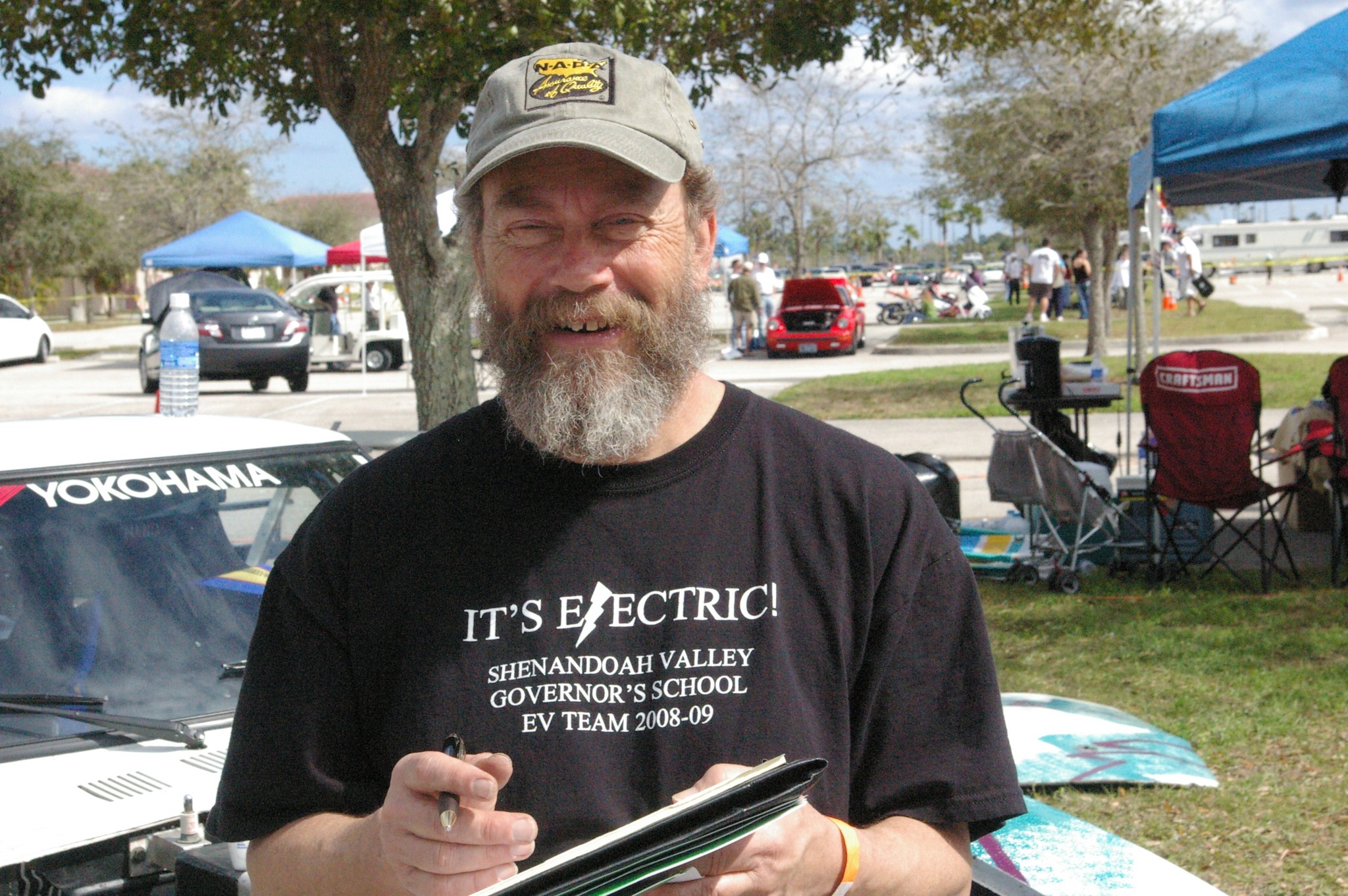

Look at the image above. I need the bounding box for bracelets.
[824,816,861,896]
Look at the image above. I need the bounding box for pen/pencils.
[436,732,466,832]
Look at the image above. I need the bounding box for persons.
[959,225,1208,322]
[920,279,940,317]
[318,286,346,371]
[363,279,395,332]
[725,252,777,358]
[203,42,1028,896]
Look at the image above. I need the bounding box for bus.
[1186,211,1348,279]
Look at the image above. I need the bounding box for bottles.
[158,293,199,420]
[1091,344,1105,384]
[1003,511,1024,531]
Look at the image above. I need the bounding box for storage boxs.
[1032,459,1114,565]
[1118,475,1214,563]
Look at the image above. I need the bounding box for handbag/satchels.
[1189,271,1213,297]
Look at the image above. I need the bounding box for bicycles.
[877,301,919,325]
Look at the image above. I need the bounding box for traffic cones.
[1162,286,1178,311]
[1337,263,1343,281]
[1230,257,1237,285]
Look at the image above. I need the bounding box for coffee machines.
[1010,335,1061,399]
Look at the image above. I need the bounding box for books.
[466,750,830,896]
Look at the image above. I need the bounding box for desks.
[997,380,1118,447]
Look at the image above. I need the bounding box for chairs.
[1139,349,1313,592]
[1307,354,1348,588]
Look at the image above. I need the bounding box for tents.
[140,211,327,294]
[711,224,751,300]
[326,240,388,272]
[1123,9,1348,573]
[360,187,460,374]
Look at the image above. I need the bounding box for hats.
[757,252,768,263]
[456,41,703,197]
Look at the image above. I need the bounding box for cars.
[766,275,868,358]
[137,270,312,396]
[0,291,52,367]
[702,251,988,302]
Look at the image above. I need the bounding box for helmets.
[743,262,753,270]
